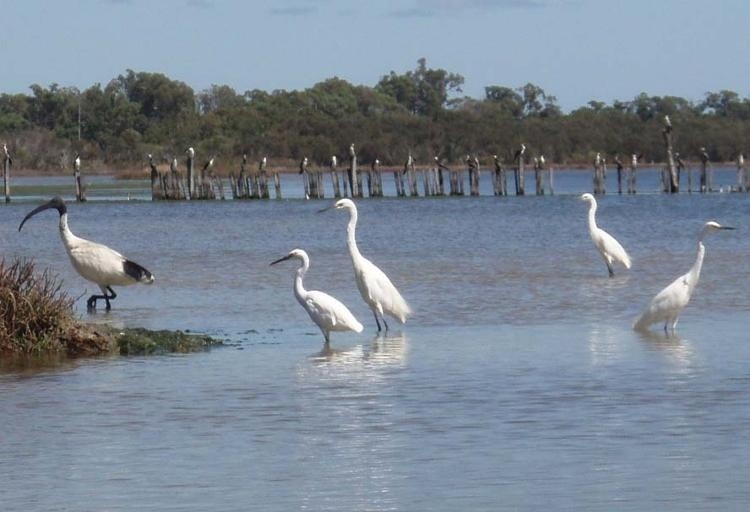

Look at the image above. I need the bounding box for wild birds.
[593,152,638,169]
[674,153,685,168]
[573,193,632,277]
[3,144,13,165]
[737,153,744,168]
[663,114,672,133]
[299,143,546,175]
[148,146,215,171]
[632,222,737,331]
[19,196,154,310]
[700,147,710,163]
[259,157,266,169]
[73,154,81,172]
[269,198,412,351]
